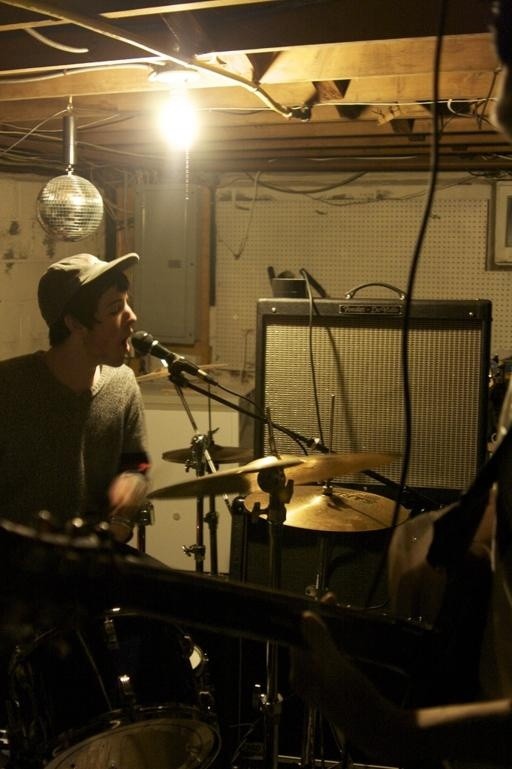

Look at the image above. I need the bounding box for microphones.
[128,326,201,381]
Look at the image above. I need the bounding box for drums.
[4,605,224,764]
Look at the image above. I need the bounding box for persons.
[284,589,512,769]
[0,251,193,761]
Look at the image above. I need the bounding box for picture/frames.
[484,179,512,271]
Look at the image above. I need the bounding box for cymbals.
[161,443,251,464]
[244,484,412,531]
[145,449,404,499]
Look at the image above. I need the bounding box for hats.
[38,252,139,326]
